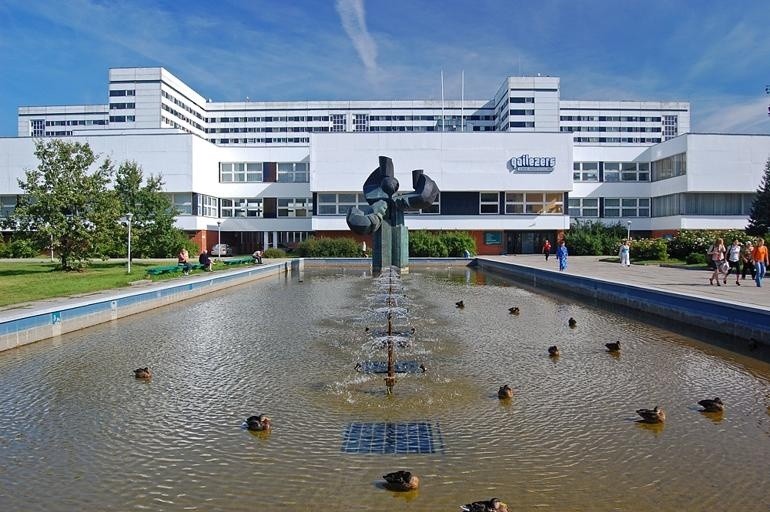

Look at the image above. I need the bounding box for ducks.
[498,384,515,398]
[634,406,667,423]
[133,366,152,378]
[568,317,578,327]
[697,397,725,412]
[245,414,271,430]
[548,346,560,356]
[508,306,519,316]
[457,496,507,512]
[383,469,419,490]
[604,340,621,351]
[455,299,465,309]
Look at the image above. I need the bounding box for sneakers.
[709,276,764,287]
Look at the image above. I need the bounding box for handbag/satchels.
[705,254,713,265]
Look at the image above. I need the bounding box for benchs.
[146,257,257,277]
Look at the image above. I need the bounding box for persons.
[252,249,263,264]
[179,248,193,275]
[556,240,568,271]
[740,241,756,280]
[752,238,769,288]
[706,238,727,286]
[619,240,630,267]
[542,239,551,262]
[199,249,217,272]
[719,239,741,286]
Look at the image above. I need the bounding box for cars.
[260,243,295,252]
[211,244,233,257]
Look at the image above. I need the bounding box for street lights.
[126,211,133,275]
[626,220,631,239]
[217,221,222,258]
